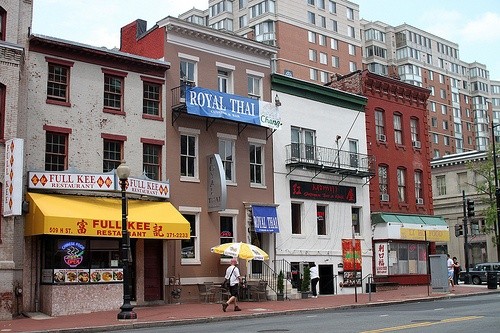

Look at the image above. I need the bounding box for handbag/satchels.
[224,279,230,290]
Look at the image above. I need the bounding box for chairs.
[198,280,270,303]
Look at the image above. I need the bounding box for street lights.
[116,158,138,319]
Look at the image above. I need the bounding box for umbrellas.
[210,241,269,275]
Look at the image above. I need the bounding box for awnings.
[25,192,191,240]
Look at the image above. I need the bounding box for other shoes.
[312,295,317,298]
[223,303,227,312]
[235,306,242,311]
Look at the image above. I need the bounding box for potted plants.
[276,270,284,301]
[301,267,310,298]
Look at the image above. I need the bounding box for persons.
[446,254,455,291]
[308,262,319,299]
[452,256,461,285]
[222,260,241,312]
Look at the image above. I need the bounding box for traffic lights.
[467,198,475,218]
[454,224,463,237]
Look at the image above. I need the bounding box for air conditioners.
[413,141,422,148]
[379,134,386,142]
[380,194,389,201]
[416,198,423,205]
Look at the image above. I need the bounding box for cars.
[459,262,500,285]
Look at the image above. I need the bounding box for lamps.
[275,101,282,107]
[275,94,279,101]
